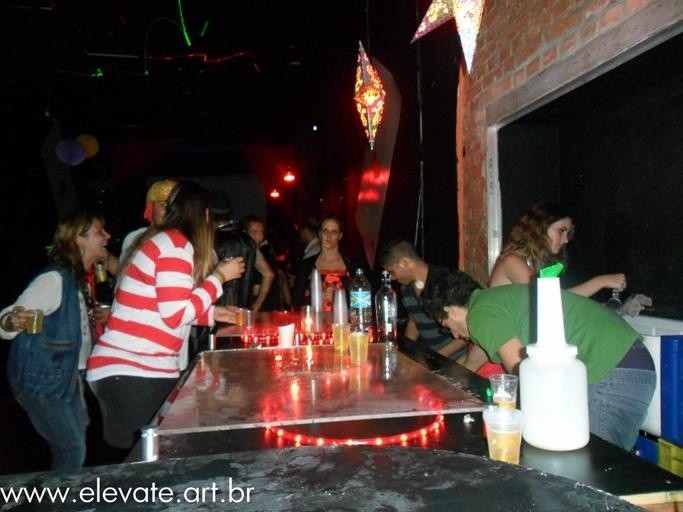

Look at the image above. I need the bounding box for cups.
[488,373,517,407]
[25,310,43,335]
[234,307,255,330]
[347,364,369,394]
[301,268,370,368]
[277,322,296,346]
[482,407,523,464]
[95,264,108,283]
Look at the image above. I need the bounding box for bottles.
[607,284,623,307]
[374,270,397,342]
[518,277,590,451]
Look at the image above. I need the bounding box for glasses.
[439,312,448,335]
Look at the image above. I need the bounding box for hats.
[148,179,178,201]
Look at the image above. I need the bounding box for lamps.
[407,0,487,78]
[350,35,389,152]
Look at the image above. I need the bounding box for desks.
[113,337,683,510]
[200,319,339,352]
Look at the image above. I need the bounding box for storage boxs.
[632,332,683,480]
